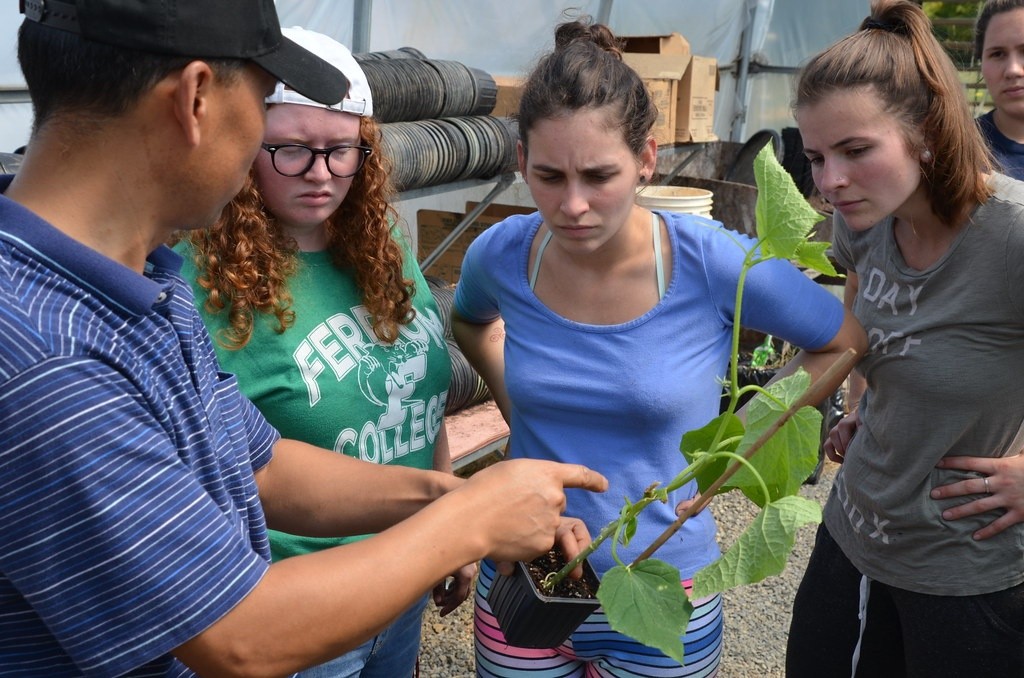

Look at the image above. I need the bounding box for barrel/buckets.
[636,185,714,221]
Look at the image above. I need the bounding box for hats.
[19,0,347,105]
[264,26,373,118]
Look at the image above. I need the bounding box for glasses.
[262,143,373,178]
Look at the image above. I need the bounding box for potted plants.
[486,139,848,668]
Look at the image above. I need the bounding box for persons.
[152,28,478,678]
[0,0,608,678]
[691,0,1024,678]
[444,21,870,678]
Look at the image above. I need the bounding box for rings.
[984,477,990,493]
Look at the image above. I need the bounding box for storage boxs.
[493,32,721,146]
